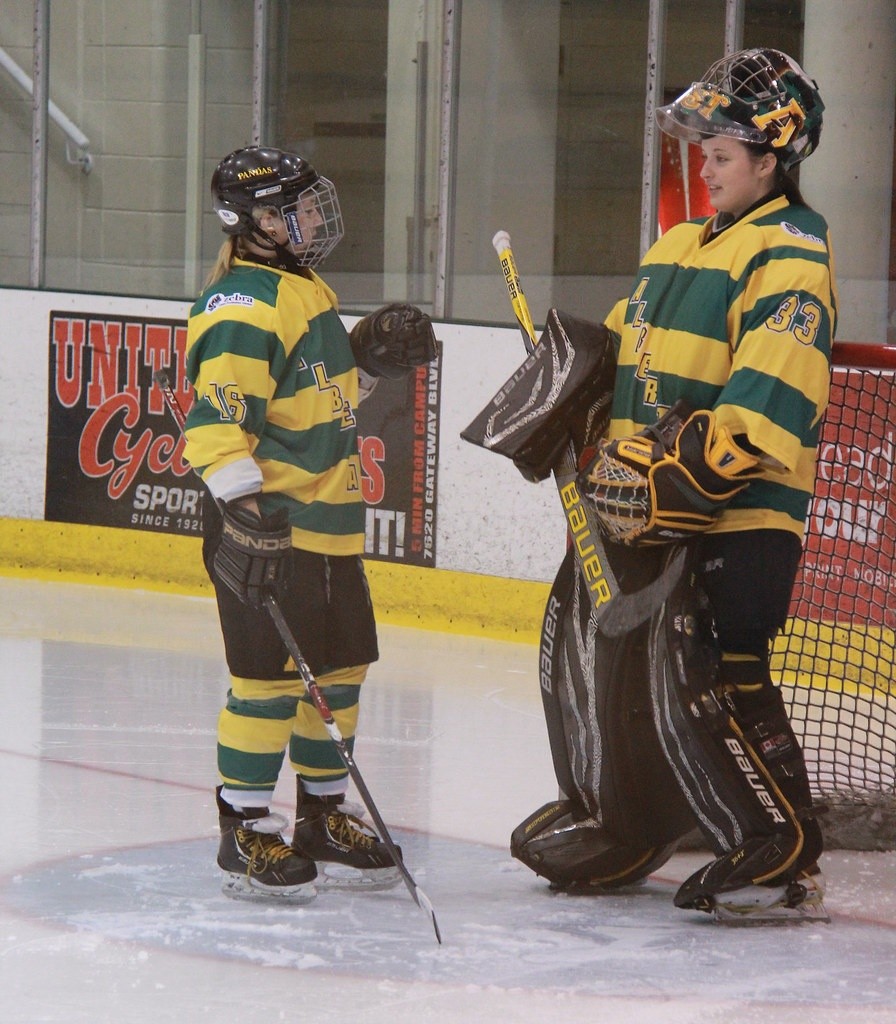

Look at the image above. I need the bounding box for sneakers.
[290,775,404,891]
[713,854,831,924]
[216,782,317,905]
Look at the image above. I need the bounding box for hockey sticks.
[153,368,443,945]
[492,229,687,639]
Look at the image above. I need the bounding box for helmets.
[675,47,827,171]
[210,144,345,266]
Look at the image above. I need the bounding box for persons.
[182,145,440,905]
[508,43,837,923]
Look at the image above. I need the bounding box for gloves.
[215,498,294,612]
[350,303,438,383]
